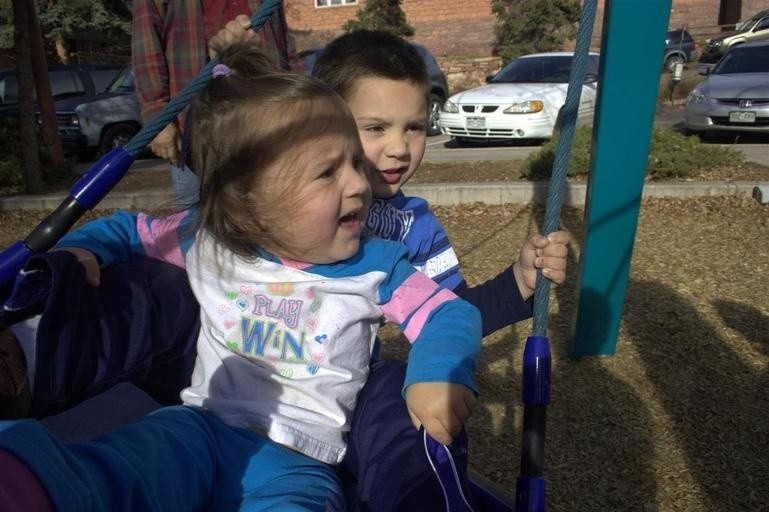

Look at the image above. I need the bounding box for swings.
[0,1,596,510]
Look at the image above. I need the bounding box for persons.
[128,2,295,211]
[1,30,572,508]
[0,40,486,511]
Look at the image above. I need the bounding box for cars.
[297,44,449,137]
[663,9,769,132]
[0,62,152,160]
[437,50,600,142]
